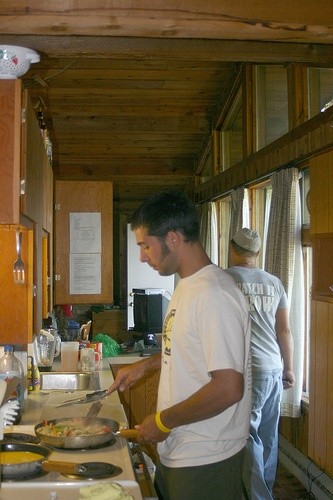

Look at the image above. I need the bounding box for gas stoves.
[0,424,135,483]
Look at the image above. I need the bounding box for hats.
[233,228,261,252]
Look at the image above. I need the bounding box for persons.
[105,196,253,500]
[224,228,294,500]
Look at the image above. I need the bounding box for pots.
[34,416,141,449]
[0,443,86,478]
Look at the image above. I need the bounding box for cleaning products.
[28,364,40,390]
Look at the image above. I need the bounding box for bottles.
[33,317,61,372]
[67,319,81,341]
[0,344,24,425]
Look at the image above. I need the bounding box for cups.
[60,341,79,372]
[79,340,103,372]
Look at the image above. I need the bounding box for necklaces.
[234,262,255,266]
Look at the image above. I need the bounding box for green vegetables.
[39,424,70,433]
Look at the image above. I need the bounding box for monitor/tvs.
[133,293,170,333]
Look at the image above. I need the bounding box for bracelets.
[155,412,172,433]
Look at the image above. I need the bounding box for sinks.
[39,371,102,392]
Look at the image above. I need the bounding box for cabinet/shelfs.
[1,79,54,233]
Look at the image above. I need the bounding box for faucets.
[40,329,55,341]
